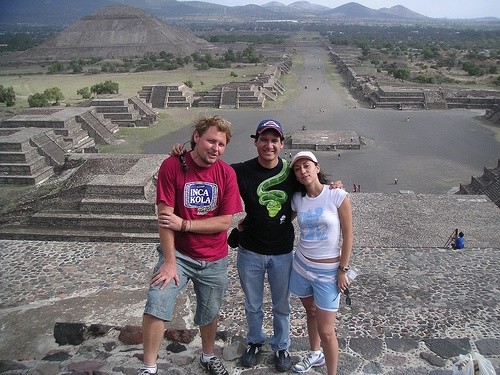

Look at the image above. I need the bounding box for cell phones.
[346,269,357,284]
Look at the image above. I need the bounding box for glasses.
[179,139,198,172]
[333,288,351,308]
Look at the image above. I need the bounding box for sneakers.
[135,363,158,375]
[241,342,262,367]
[274,349,292,371]
[199,353,229,375]
[291,352,325,373]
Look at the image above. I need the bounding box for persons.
[284,152,291,158]
[353,184,360,192]
[393,177,399,184]
[138,114,242,375]
[288,151,353,375]
[170,120,345,372]
[337,153,340,159]
[452,232,464,248]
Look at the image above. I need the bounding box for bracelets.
[180,220,191,231]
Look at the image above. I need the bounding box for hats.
[250,119,286,141]
[289,150,318,168]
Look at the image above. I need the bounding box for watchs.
[338,265,350,272]
